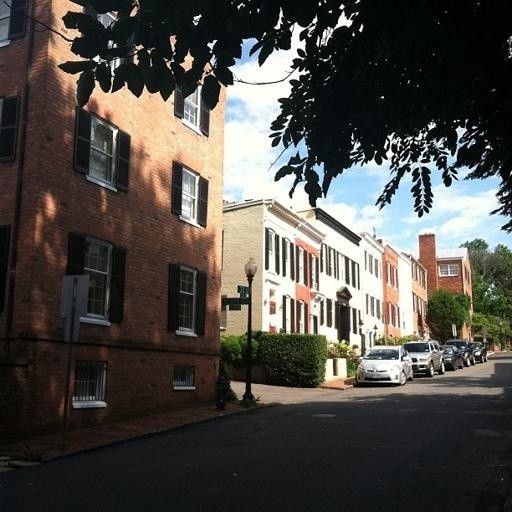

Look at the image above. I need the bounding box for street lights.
[244,257,260,406]
[498,321,503,351]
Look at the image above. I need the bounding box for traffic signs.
[237,285,250,294]
[222,297,249,305]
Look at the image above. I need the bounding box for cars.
[356,338,488,386]
